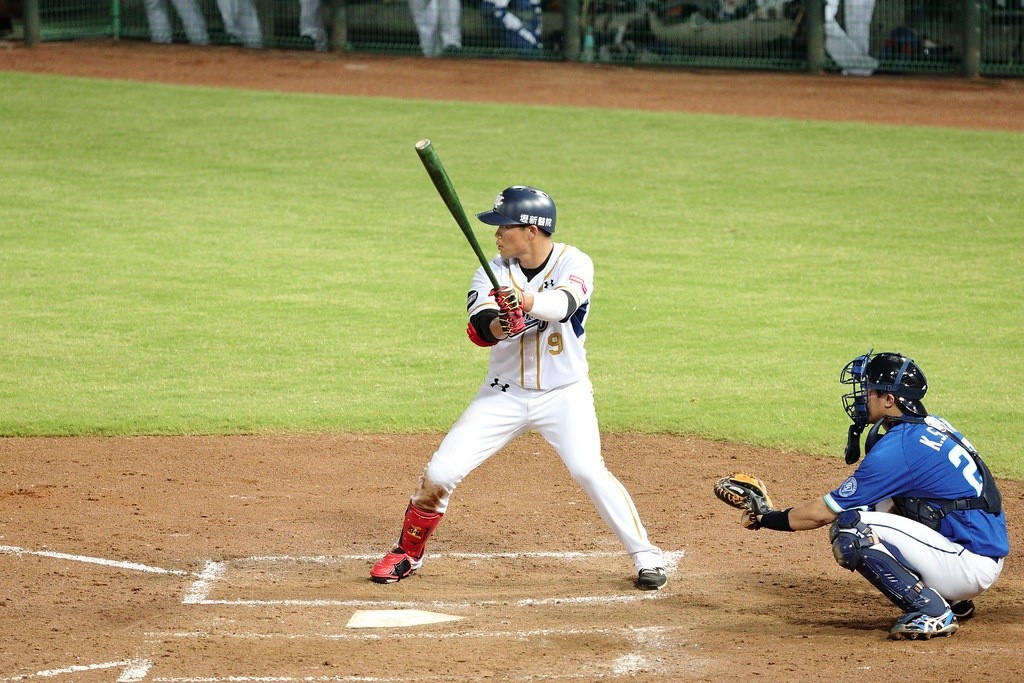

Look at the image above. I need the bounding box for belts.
[992,556,1000,563]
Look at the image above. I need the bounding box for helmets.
[867,353,929,416]
[474,185,556,233]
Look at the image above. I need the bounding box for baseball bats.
[414,137,501,290]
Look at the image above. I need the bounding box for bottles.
[582,25,596,62]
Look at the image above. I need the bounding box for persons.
[141,0,879,77]
[739,349,1009,640]
[369,185,669,590]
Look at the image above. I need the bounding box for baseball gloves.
[712,472,775,532]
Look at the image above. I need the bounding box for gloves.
[488,287,525,313]
[496,309,525,334]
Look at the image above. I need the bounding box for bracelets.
[760,507,795,532]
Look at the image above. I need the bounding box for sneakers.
[952,599,977,623]
[638,566,667,591]
[892,605,960,640]
[373,550,417,583]
